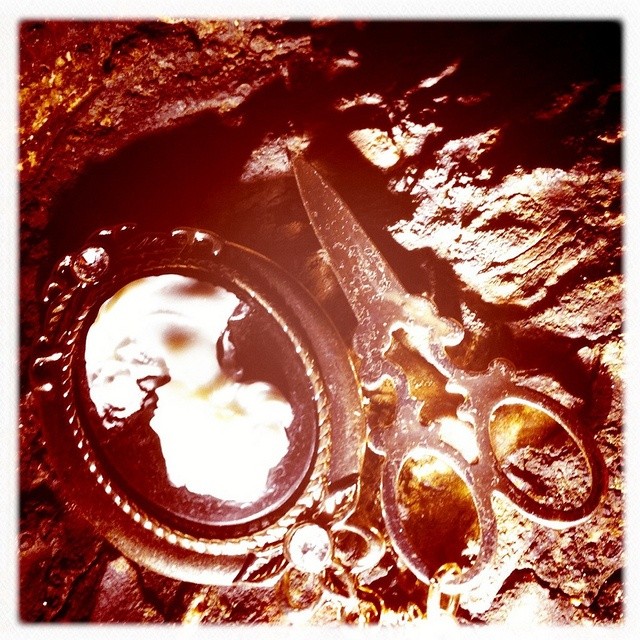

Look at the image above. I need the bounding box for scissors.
[291,156,605,587]
